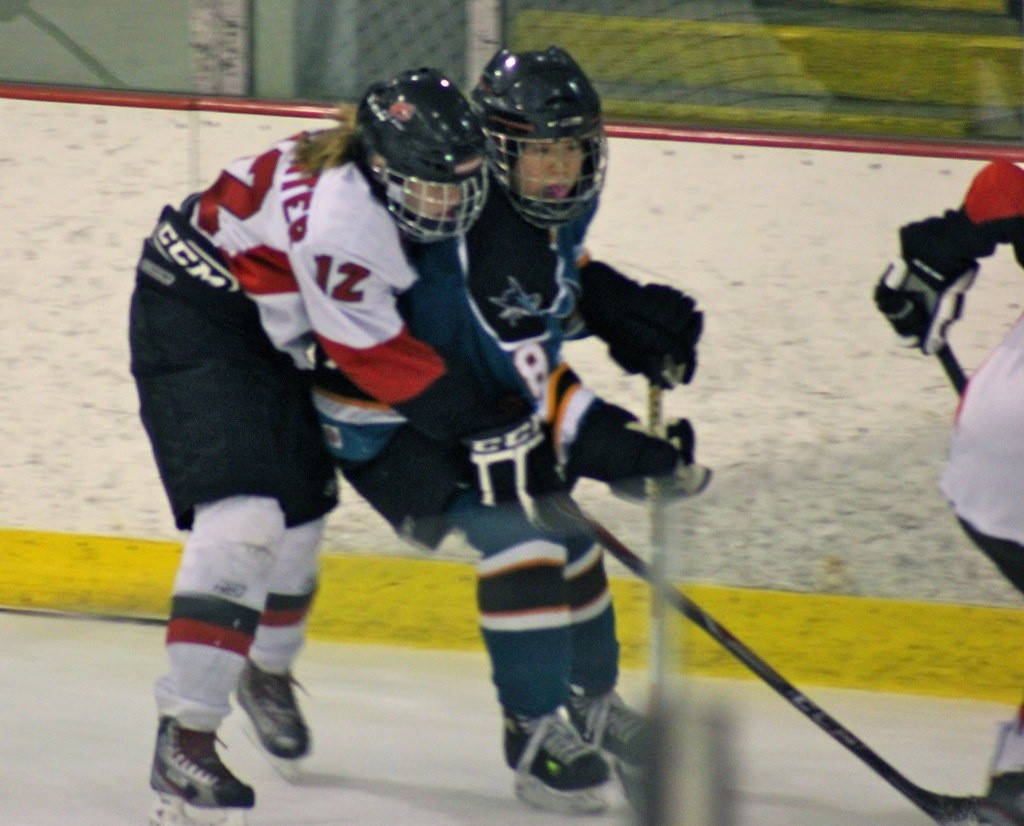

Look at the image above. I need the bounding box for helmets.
[354,67,489,241]
[472,44,602,225]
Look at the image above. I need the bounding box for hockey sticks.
[631,375,679,826]
[560,495,1024,826]
[935,334,975,398]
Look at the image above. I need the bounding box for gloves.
[607,284,706,388]
[873,212,989,359]
[568,397,711,502]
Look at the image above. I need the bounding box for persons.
[127,41,710,826]
[872,156,1024,825]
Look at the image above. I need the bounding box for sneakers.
[564,693,658,777]
[503,705,610,814]
[148,715,256,825]
[227,658,316,783]
